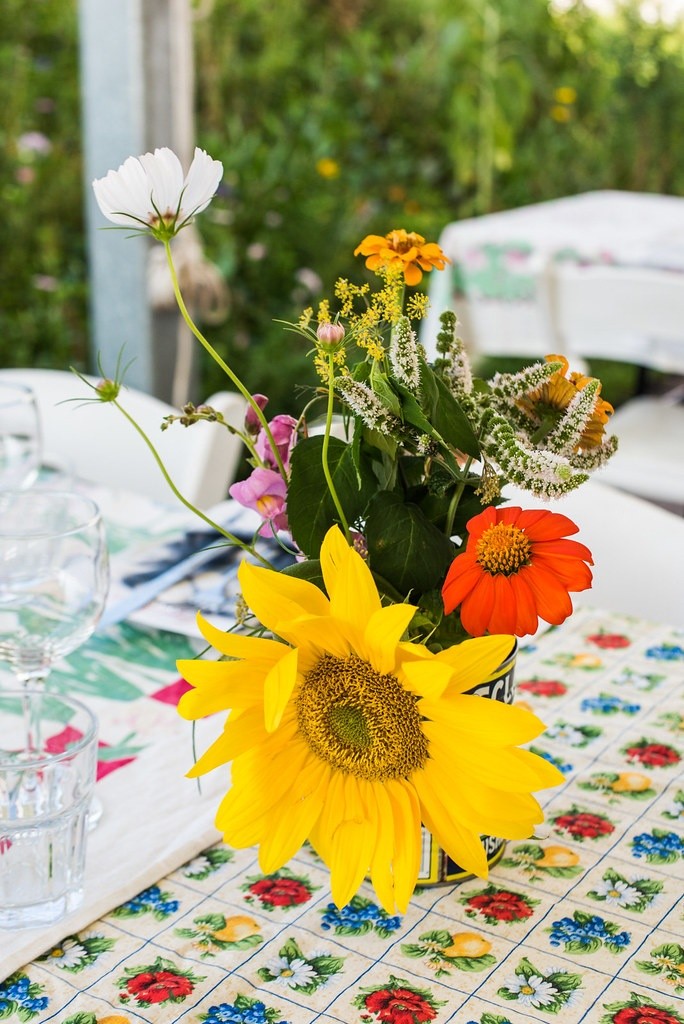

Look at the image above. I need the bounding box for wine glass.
[0,488,111,841]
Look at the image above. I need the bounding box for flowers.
[54,140,621,920]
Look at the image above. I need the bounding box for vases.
[363,637,520,893]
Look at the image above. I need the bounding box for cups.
[0,690,100,933]
[0,378,45,494]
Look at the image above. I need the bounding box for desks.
[0,191,683,1024]
